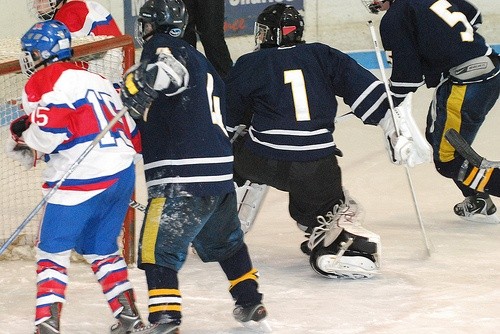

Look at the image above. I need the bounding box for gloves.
[385,131,414,166]
[7,112,42,171]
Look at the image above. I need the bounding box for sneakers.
[232,302,273,334]
[32,318,60,334]
[110,305,146,334]
[297,199,380,255]
[309,226,377,279]
[453,192,497,216]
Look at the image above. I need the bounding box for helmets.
[359,0,395,14]
[134,0,189,48]
[130,323,183,334]
[253,3,305,50]
[19,19,74,78]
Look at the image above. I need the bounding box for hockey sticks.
[129,198,147,213]
[0,106,130,253]
[368,21,431,256]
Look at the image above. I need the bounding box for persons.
[120,0,268,334]
[182,0,233,75]
[27,0,124,96]
[7,20,146,334]
[218,3,414,280]
[361,0,500,223]
